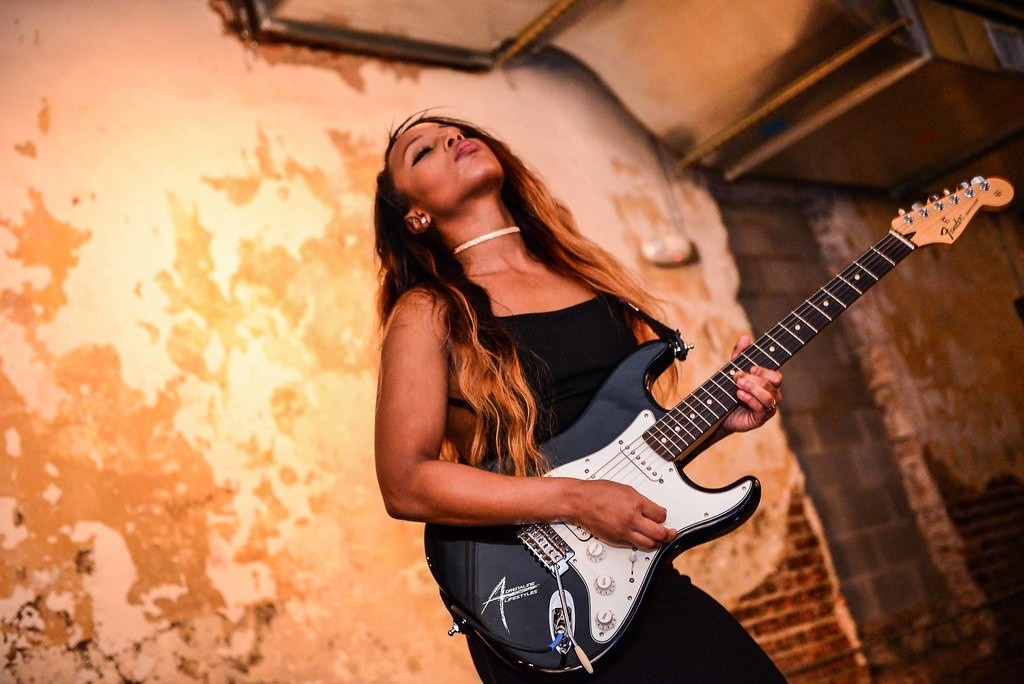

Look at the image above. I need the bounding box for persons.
[374,108,791,684]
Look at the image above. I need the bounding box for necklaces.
[447,225,521,255]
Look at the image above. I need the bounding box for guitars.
[424,177,1014,676]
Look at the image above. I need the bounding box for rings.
[765,400,777,413]
